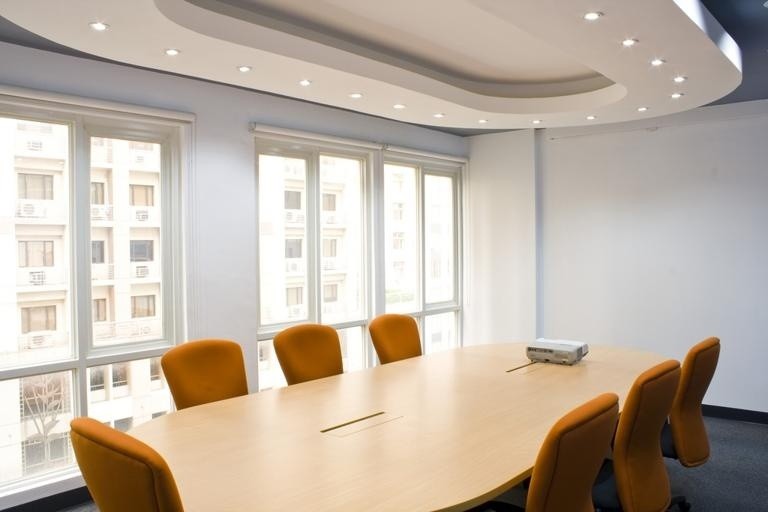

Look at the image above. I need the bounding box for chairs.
[162,338,250,409]
[368,315,422,364]
[70,417,184,512]
[468,394,620,512]
[274,323,344,385]
[611,336,721,466]
[524,359,692,512]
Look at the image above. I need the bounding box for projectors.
[525,338,589,366]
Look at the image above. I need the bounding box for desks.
[126,342,665,510]
